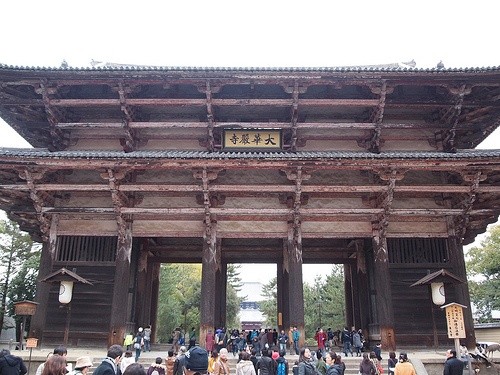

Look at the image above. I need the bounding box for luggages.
[125,346,132,358]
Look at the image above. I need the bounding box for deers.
[485,343,500,357]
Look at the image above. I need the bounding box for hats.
[139,328,143,332]
[272,352,279,359]
[184,347,208,369]
[277,357,285,362]
[75,356,93,368]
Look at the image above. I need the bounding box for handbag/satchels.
[191,337,196,342]
[218,341,223,345]
[362,347,366,352]
[144,336,151,341]
[344,334,351,340]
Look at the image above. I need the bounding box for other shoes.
[234,352,236,356]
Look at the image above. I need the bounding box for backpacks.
[151,371,159,375]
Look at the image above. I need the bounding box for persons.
[394,352,417,375]
[293,347,346,375]
[0,348,27,375]
[359,343,385,375]
[387,352,399,375]
[205,327,278,355]
[235,346,290,375]
[442,349,463,375]
[93,345,123,375]
[165,345,231,375]
[187,327,196,350]
[277,330,289,349]
[147,357,166,375]
[121,325,152,372]
[123,363,147,375]
[36,346,70,375]
[292,326,300,354]
[171,328,180,355]
[66,357,93,375]
[316,326,364,356]
